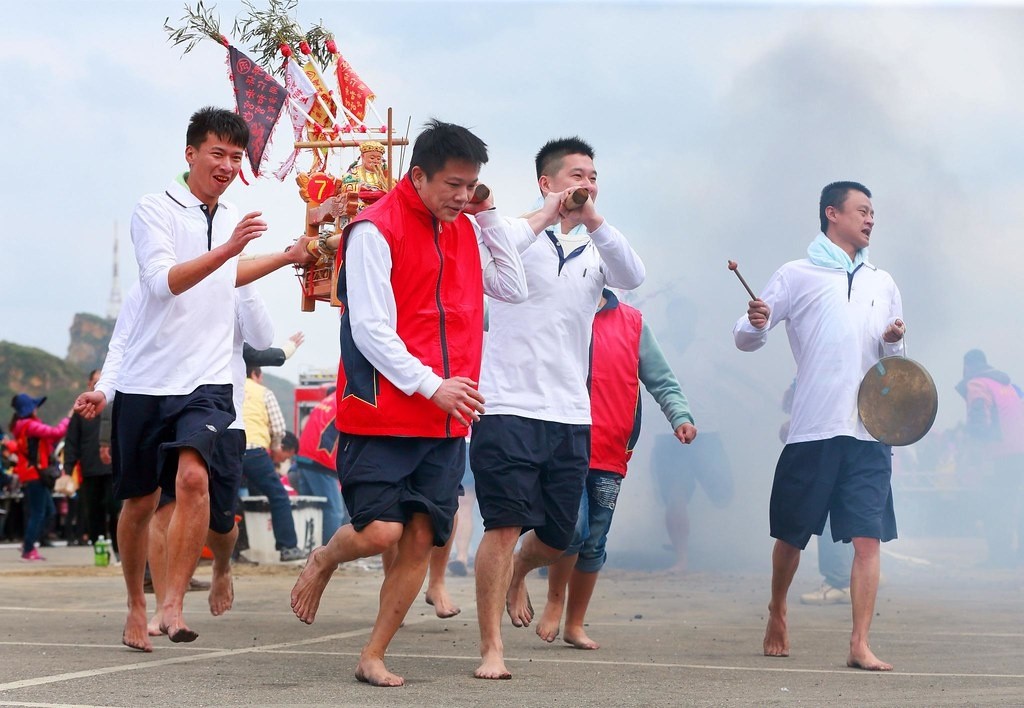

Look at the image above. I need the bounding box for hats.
[12,393,47,417]
[964,348,987,362]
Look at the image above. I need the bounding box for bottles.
[106,539,116,565]
[95,535,108,566]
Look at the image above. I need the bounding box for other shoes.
[144,580,154,593]
[40,544,54,547]
[185,578,211,591]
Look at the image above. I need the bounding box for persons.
[470,136,647,676]
[116,106,266,652]
[2,296,1024,618]
[734,182,905,671]
[291,118,528,687]
[537,288,696,654]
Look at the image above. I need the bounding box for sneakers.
[878,574,887,589]
[800,580,852,605]
[19,546,47,560]
[280,546,307,561]
[231,554,258,567]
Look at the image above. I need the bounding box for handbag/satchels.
[38,463,62,490]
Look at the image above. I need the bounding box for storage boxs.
[241,495,328,559]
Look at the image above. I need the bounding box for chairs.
[301,202,341,311]
